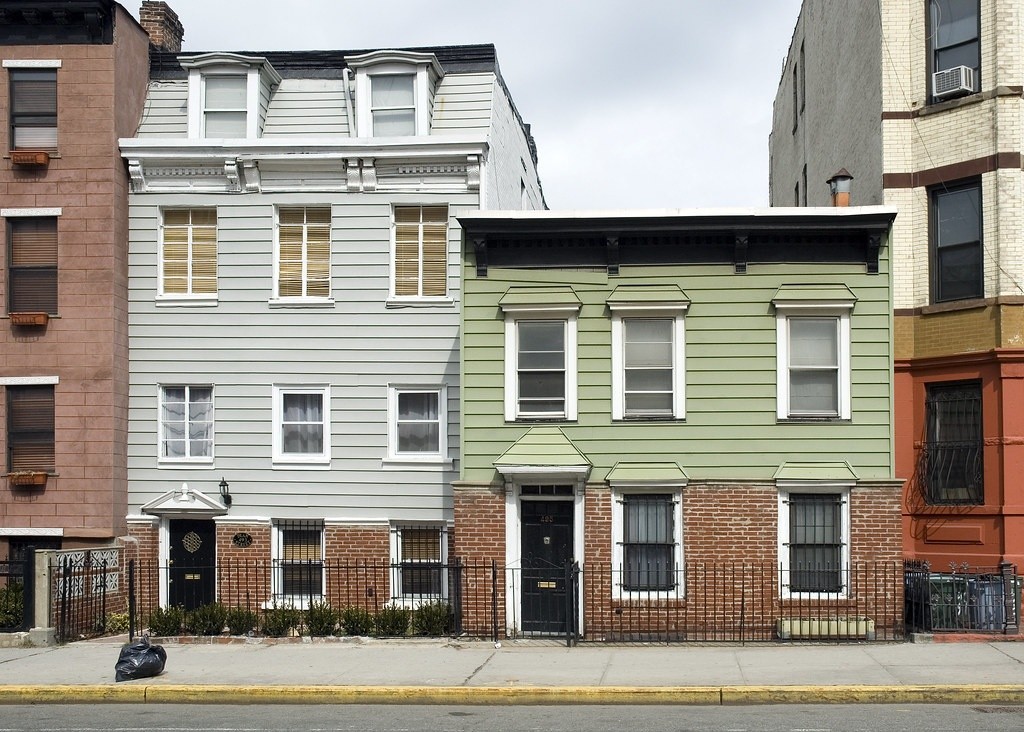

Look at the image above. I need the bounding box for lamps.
[219,477,231,504]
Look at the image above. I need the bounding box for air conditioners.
[932,64,973,100]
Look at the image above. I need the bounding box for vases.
[8,151,49,165]
[777,617,875,640]
[7,469,48,485]
[7,312,49,326]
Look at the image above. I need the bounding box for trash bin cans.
[905,572,1023,635]
